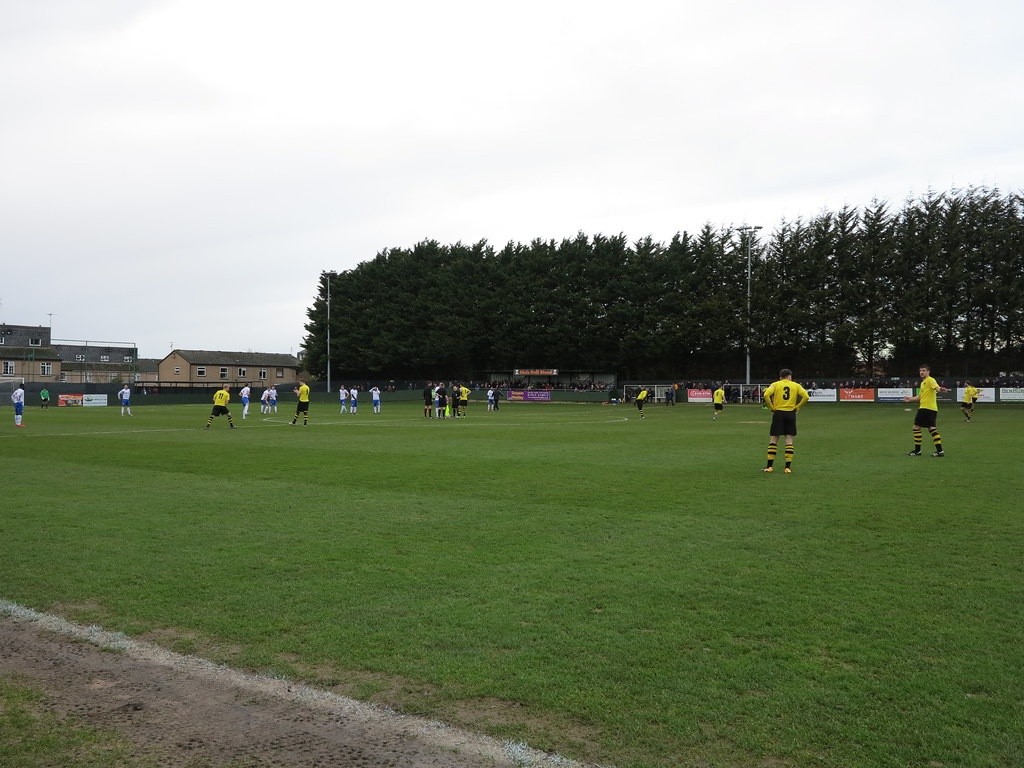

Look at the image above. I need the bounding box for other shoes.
[966,420,970,423]
[204,426,209,430]
[120,414,124,417]
[338,402,645,422]
[15,424,25,428]
[304,425,307,427]
[242,418,247,420]
[46,409,48,410]
[40,408,43,410]
[715,413,718,418]
[762,406,769,410]
[713,418,716,421]
[288,421,295,426]
[129,414,134,417]
[964,417,968,420]
[231,426,238,430]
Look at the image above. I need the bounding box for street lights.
[745,226,763,385]
[323,272,337,393]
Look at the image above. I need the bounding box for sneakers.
[930,450,944,457]
[906,449,922,456]
[784,468,791,474]
[760,467,773,473]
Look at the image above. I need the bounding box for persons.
[289,378,310,427]
[954,381,963,387]
[340,384,358,414]
[940,380,947,388]
[665,387,674,406]
[423,381,471,420]
[487,387,503,412]
[140,386,158,395]
[383,385,396,392]
[798,380,919,390]
[468,379,607,389]
[623,386,653,419]
[903,364,948,457]
[978,378,1024,388]
[239,383,250,420]
[40,387,50,410]
[960,381,978,423]
[117,384,133,417]
[11,384,26,427]
[260,386,278,414]
[369,386,381,414]
[724,380,770,409]
[713,386,727,421]
[761,369,810,473]
[674,380,721,391]
[205,384,238,429]
